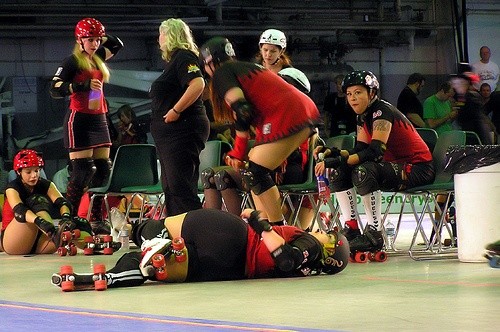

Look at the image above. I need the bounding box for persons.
[471,46,500,96]
[201,35,322,226]
[55,207,352,289]
[103,98,119,141]
[201,66,312,218]
[312,69,437,260]
[1,149,122,257]
[479,83,497,122]
[49,16,125,234]
[326,74,358,137]
[426,192,457,248]
[145,17,213,217]
[396,72,430,129]
[116,104,149,145]
[424,82,460,153]
[250,28,295,74]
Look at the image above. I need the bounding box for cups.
[88,89,101,110]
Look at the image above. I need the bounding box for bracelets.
[172,107,181,116]
[448,113,452,119]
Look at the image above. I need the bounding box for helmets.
[277,67,311,94]
[258,29,287,50]
[75,18,106,38]
[13,149,45,170]
[199,37,236,63]
[340,70,380,98]
[317,230,350,274]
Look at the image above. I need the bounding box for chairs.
[6,129,482,262]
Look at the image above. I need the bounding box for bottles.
[121,221,130,251]
[385,219,396,249]
[315,153,331,198]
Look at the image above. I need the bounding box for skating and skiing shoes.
[349,224,388,262]
[137,236,188,280]
[81,234,122,256]
[50,219,81,256]
[50,261,107,292]
[338,227,361,241]
